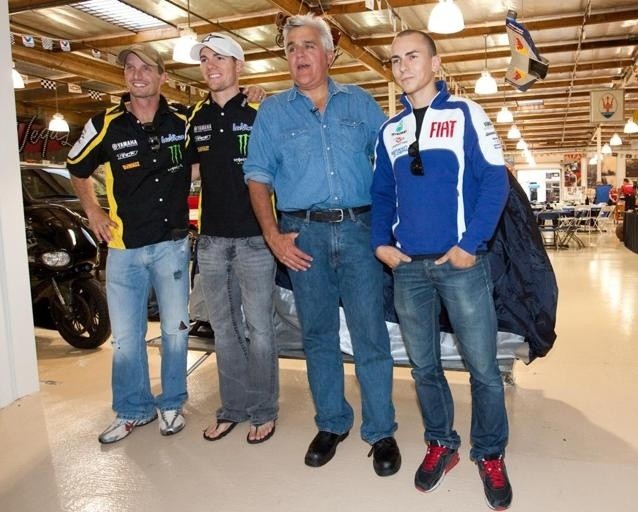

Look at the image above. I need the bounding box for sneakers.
[474,448,513,512]
[97,403,158,444]
[413,439,461,493]
[157,404,186,436]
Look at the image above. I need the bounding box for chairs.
[529,198,616,253]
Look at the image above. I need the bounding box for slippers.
[245,418,276,445]
[203,416,240,442]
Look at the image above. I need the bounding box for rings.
[259,96,262,99]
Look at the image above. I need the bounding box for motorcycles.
[25,199,110,348]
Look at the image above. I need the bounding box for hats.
[189,30,246,62]
[114,42,167,72]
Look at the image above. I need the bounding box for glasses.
[408,139,425,179]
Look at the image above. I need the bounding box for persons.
[66,44,267,444]
[616,178,636,211]
[370,29,512,511]
[242,16,400,476]
[185,32,280,444]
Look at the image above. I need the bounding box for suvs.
[20,164,194,322]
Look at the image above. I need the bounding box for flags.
[10,33,204,105]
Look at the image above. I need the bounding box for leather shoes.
[366,434,402,478]
[302,425,350,467]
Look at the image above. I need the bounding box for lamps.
[428,0,638,186]
[48,83,69,133]
[12,61,25,90]
[172,0,203,66]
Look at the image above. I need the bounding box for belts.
[280,203,375,223]
